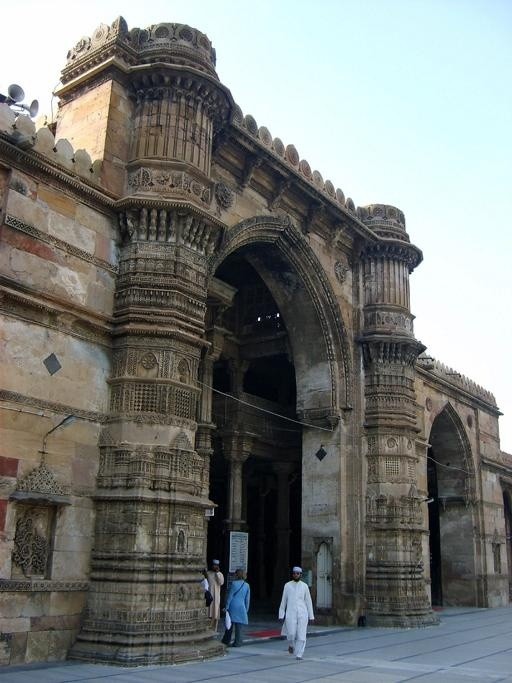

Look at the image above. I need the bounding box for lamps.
[40,414,77,454]
[418,498,435,508]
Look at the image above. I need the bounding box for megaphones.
[5,83,25,106]
[19,100,39,117]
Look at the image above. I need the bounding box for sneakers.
[289,645,293,654]
[296,657,303,660]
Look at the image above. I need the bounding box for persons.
[278,565,316,660]
[207,558,225,621]
[221,568,252,647]
[200,569,210,592]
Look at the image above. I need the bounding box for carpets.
[246,630,282,638]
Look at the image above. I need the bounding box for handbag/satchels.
[222,608,232,631]
[204,590,213,607]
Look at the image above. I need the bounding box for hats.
[293,566,302,573]
[212,559,220,565]
